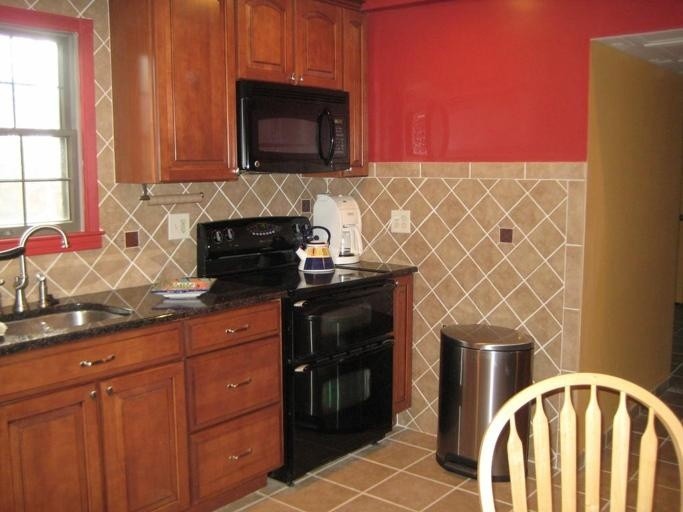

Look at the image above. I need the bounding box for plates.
[150,296,205,313]
[146,278,217,301]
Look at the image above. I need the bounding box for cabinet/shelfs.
[108,0,240,185]
[184,299,284,506]
[234,0,343,90]
[392,273,412,414]
[303,0,368,179]
[0,321,191,512]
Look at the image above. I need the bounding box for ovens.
[267,282,393,488]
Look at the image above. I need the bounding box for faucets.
[14,224,71,313]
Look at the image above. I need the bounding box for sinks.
[4,309,129,338]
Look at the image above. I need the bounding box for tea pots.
[292,224,333,278]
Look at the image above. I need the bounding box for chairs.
[476,371,683,512]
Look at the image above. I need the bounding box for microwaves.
[235,78,353,175]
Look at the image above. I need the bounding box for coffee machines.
[311,193,364,264]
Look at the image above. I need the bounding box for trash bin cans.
[436,325,535,482]
